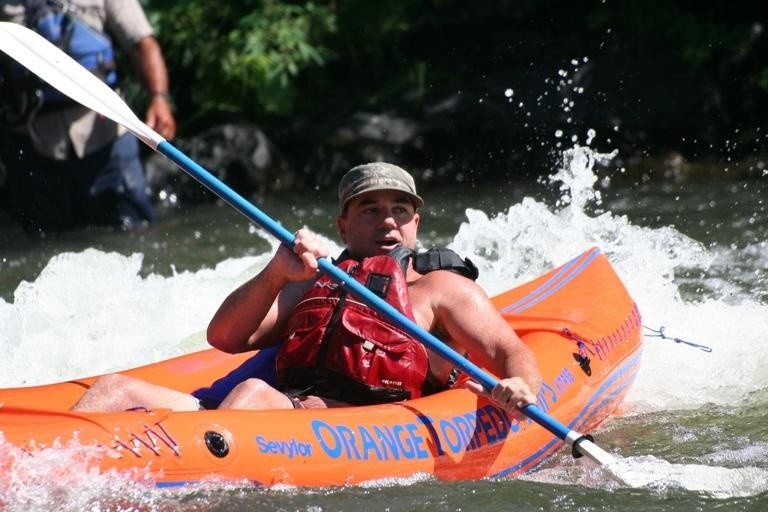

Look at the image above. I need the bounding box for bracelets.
[152,92,168,101]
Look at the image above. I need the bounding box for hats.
[338,161,424,217]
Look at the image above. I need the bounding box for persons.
[0,0,176,230]
[68,161,542,416]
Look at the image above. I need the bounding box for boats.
[1,246,642,504]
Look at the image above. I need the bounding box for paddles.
[0,21,616,465]
